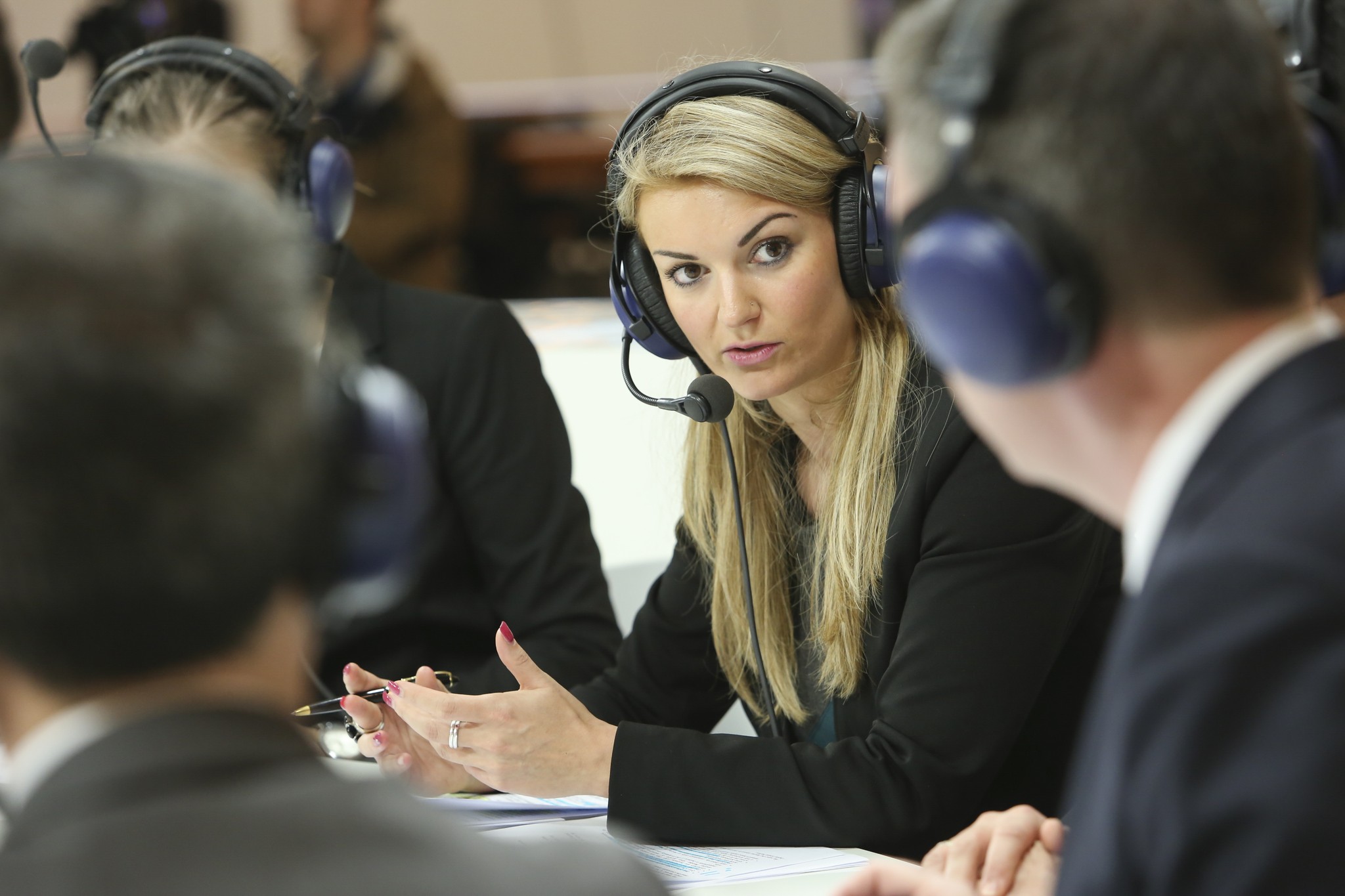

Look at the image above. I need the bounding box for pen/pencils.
[291,670,461,717]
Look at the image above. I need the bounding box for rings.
[449,720,461,748]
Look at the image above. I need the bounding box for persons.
[0,11,23,149]
[339,56,1122,865]
[286,1,469,293]
[832,1,1344,895]
[83,34,623,763]
[64,0,229,85]
[0,147,676,895]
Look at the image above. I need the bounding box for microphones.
[620,328,735,424]
[17,38,61,161]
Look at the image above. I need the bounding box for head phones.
[81,33,353,250]
[894,2,1101,392]
[602,60,899,367]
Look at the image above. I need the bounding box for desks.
[323,759,920,896]
